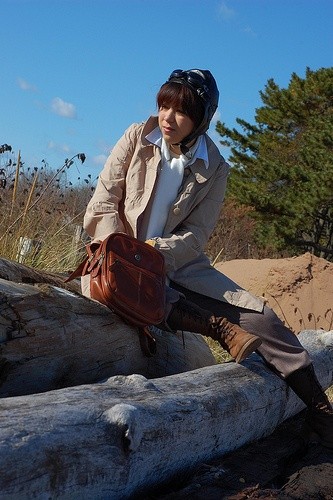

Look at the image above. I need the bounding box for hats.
[168,69,219,145]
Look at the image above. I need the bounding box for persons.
[81,70,333,442]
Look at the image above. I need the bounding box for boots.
[185,301,262,363]
[285,363,333,444]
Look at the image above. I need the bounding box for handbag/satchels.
[64,233,164,353]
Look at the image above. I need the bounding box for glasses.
[168,70,213,108]
